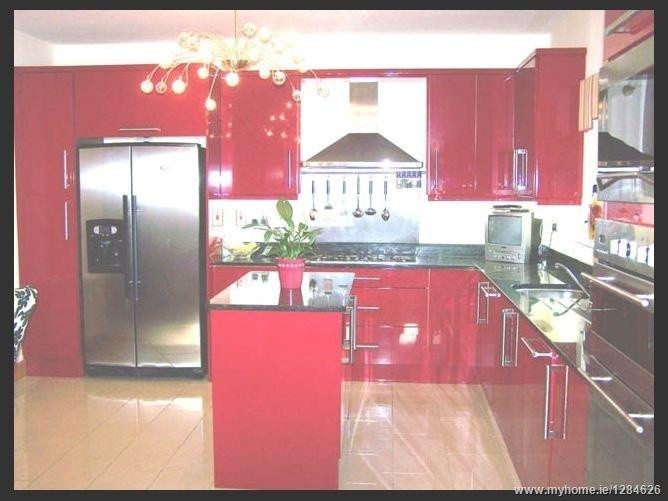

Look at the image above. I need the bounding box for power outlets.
[550,218,560,234]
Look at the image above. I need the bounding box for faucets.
[552,257,590,300]
[551,298,581,318]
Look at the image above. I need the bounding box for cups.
[322,279,334,295]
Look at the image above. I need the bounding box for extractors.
[303,76,423,169]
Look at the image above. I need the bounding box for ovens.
[574,35,654,490]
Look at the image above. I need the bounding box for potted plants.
[241,200,325,292]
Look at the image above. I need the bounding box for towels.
[577,70,591,133]
[588,72,598,120]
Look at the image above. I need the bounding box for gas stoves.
[310,244,416,263]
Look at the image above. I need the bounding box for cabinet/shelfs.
[602,9,653,60]
[474,273,504,421]
[221,73,301,202]
[15,65,79,383]
[74,67,211,142]
[541,355,585,491]
[426,72,518,205]
[428,270,486,367]
[350,266,426,365]
[493,309,551,491]
[502,49,586,207]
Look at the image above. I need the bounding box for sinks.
[511,282,588,302]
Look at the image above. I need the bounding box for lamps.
[132,11,333,116]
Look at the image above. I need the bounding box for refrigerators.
[79,139,205,372]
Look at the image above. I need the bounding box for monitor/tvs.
[485,211,534,264]
